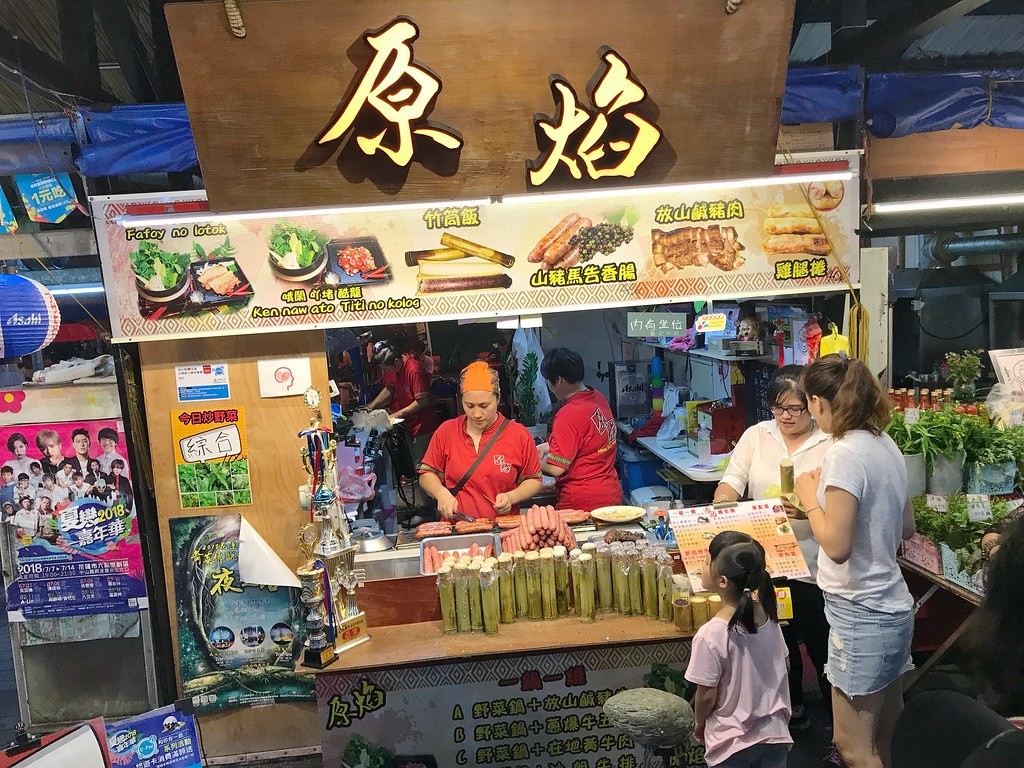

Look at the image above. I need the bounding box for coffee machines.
[334,434,362,501]
[596,361,673,420]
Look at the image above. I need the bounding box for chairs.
[381,423,421,531]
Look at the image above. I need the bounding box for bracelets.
[979,537,1001,561]
[804,507,822,516]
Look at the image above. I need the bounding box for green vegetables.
[265,223,331,267]
[177,459,251,509]
[129,233,233,289]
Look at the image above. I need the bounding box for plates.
[190,258,254,302]
[592,505,647,522]
[327,236,394,286]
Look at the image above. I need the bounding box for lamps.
[20,268,104,293]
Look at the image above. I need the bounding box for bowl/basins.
[269,240,325,275]
[135,262,186,298]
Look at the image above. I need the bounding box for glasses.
[769,406,807,417]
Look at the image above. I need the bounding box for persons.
[413,341,435,377]
[363,341,439,529]
[483,340,510,364]
[0,426,133,546]
[891,502,1024,767]
[792,351,917,768]
[537,345,622,512]
[684,530,796,768]
[417,361,542,526]
[712,365,847,768]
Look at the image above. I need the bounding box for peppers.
[226,282,254,296]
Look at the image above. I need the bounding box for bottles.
[697,422,711,465]
[885,388,979,412]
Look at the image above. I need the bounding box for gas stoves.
[335,421,378,456]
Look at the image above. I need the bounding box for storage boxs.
[940,542,985,596]
[696,400,745,455]
[928,451,963,494]
[629,486,674,507]
[617,443,665,500]
[901,532,942,575]
[708,338,736,351]
[966,462,1015,495]
[902,453,926,496]
[684,431,698,458]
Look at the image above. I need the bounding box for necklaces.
[809,420,816,432]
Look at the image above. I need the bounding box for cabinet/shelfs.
[614,335,773,481]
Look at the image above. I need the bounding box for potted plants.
[503,349,547,438]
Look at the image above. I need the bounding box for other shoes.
[823,715,833,730]
[787,704,811,729]
[401,510,434,528]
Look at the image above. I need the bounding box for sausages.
[527,212,593,269]
[415,504,593,574]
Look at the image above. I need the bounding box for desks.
[294,613,707,768]
[896,556,985,695]
[457,362,505,416]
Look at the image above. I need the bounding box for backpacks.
[385,423,418,478]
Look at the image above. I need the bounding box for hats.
[373,339,389,354]
[381,351,401,364]
[377,347,389,360]
[459,358,501,395]
